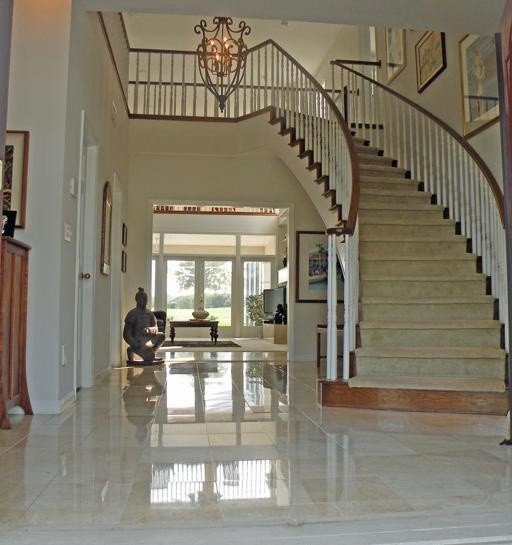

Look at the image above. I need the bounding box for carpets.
[161,341,242,347]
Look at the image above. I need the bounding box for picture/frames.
[458,33,501,138]
[296,230,346,302]
[385,27,407,86]
[122,251,127,272]
[0,129,29,229]
[101,181,113,275]
[123,224,128,246]
[415,31,447,92]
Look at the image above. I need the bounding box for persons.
[122,287,165,362]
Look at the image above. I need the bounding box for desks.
[170,321,219,343]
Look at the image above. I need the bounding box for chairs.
[153,311,166,333]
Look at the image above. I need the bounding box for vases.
[192,308,209,319]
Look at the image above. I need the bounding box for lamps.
[194,17,251,112]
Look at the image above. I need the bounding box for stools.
[316,324,358,367]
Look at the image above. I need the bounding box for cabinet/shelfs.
[0,235,36,429]
[262,324,287,344]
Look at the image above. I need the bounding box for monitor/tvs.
[264,288,285,313]
